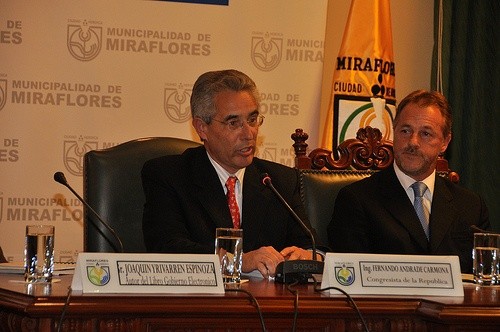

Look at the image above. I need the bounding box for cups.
[24,225,55,283]
[473,233,500,286]
[215,228,242,289]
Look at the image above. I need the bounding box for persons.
[327,89,494,276]
[141,69,333,279]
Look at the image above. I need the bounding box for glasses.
[194,112,266,130]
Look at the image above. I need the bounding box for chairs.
[290,125,459,237]
[83,137,202,254]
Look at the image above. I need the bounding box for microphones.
[54,171,125,252]
[258,172,325,285]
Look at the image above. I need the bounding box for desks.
[0,272,500,332]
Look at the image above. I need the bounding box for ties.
[225,177,240,230]
[410,181,430,241]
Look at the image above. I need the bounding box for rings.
[302,258,306,260]
